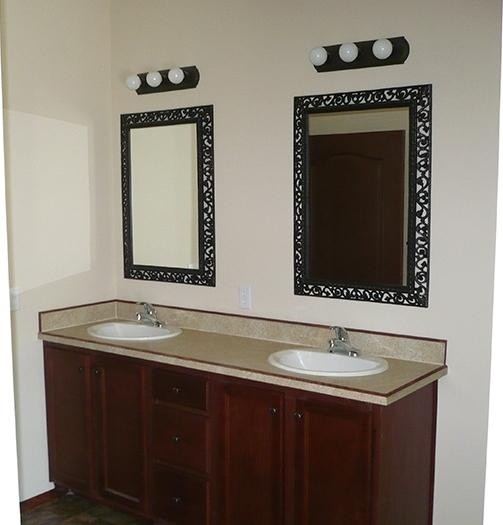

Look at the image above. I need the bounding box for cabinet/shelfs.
[218,376,433,525]
[42,341,149,515]
[148,365,216,524]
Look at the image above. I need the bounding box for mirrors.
[118,103,218,286]
[294,83,431,309]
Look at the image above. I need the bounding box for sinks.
[267,347,391,377]
[87,319,183,342]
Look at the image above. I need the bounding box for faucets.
[135,301,167,328]
[328,326,359,356]
